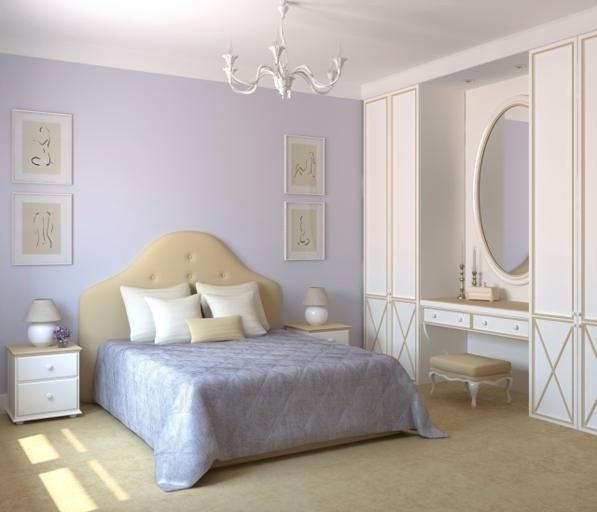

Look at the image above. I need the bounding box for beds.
[78,229,413,473]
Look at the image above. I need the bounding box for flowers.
[51,325,73,342]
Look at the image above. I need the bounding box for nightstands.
[286,322,353,347]
[5,345,84,425]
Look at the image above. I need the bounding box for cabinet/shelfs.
[358,34,597,438]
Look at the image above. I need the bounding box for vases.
[57,340,67,348]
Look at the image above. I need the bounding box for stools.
[426,352,514,409]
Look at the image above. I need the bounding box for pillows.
[119,280,272,347]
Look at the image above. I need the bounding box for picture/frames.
[283,134,327,262]
[10,108,74,267]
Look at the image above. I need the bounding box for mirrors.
[473,102,534,285]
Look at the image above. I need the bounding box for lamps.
[220,0,349,102]
[25,298,62,350]
